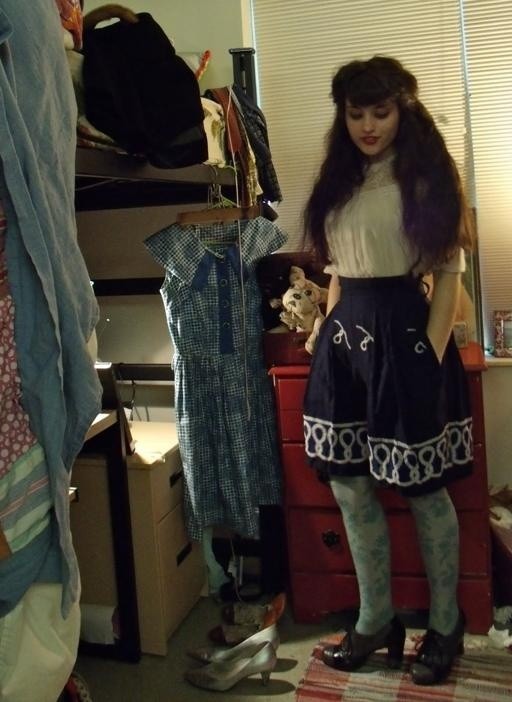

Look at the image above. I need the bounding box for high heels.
[411,609,465,686]
[182,592,288,691]
[322,614,406,671]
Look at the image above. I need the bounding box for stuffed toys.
[270,265,328,355]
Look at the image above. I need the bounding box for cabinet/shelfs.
[151,439,210,659]
[265,331,497,634]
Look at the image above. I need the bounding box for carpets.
[290,630,511,702]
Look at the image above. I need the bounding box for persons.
[302,55,473,681]
